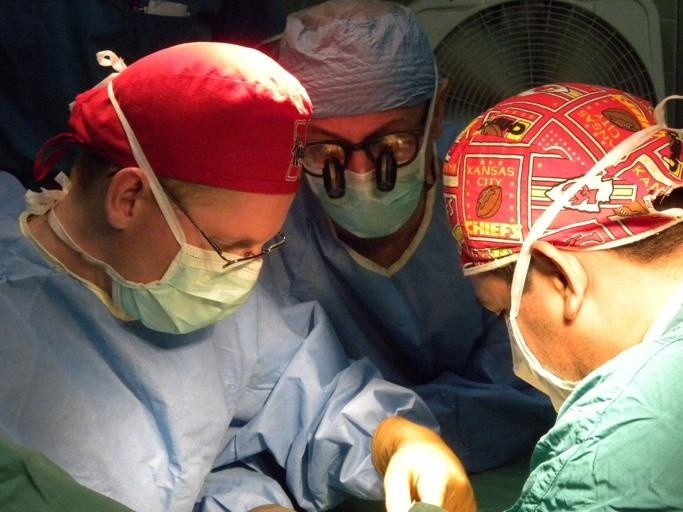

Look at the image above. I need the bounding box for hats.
[276,0,443,119]
[67,39,312,196]
[443,81,683,276]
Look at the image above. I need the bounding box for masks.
[299,77,443,240]
[505,219,584,416]
[106,168,264,336]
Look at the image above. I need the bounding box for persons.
[438,82,683,511]
[0,38,483,510]
[239,2,562,479]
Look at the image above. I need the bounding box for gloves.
[372,416,478,512]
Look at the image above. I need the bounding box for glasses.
[161,168,287,270]
[293,130,426,179]
